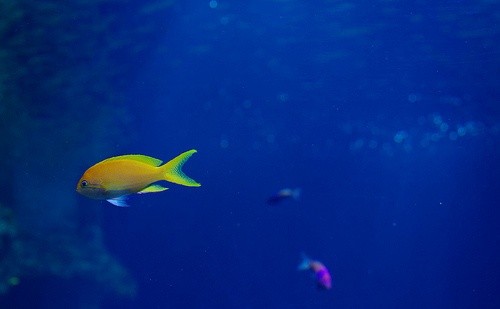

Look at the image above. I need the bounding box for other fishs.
[296,252,332,291]
[75,149,202,207]
[265,187,302,206]
